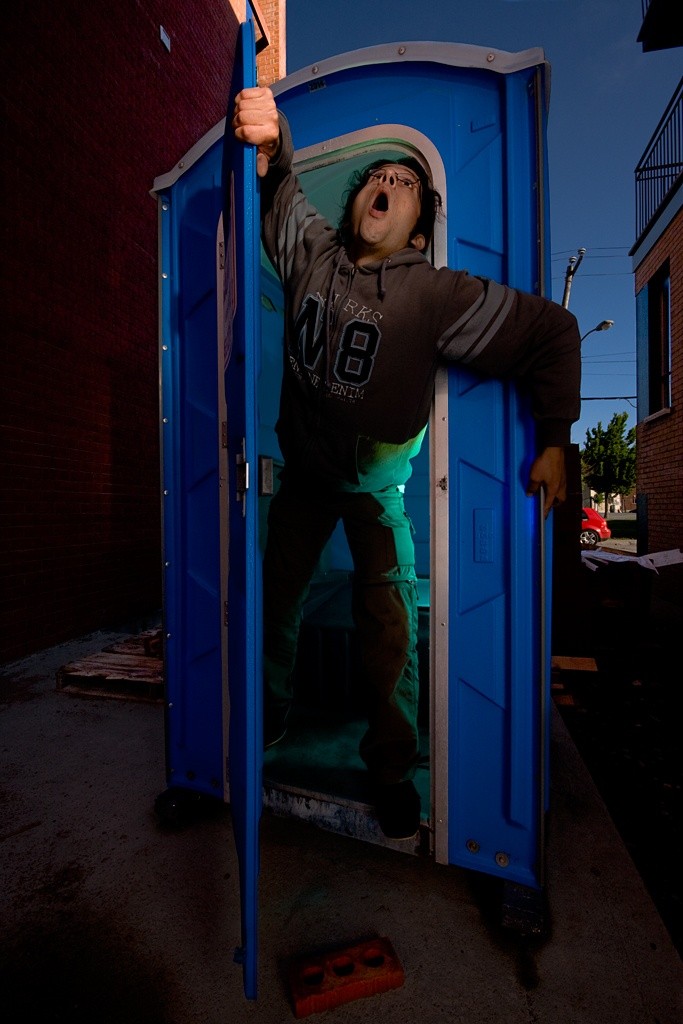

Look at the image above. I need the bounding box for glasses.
[368,167,422,202]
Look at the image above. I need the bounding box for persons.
[234,86,582,838]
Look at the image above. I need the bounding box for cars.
[581,507,611,550]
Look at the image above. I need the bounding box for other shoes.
[374,775,421,840]
[263,702,292,748]
